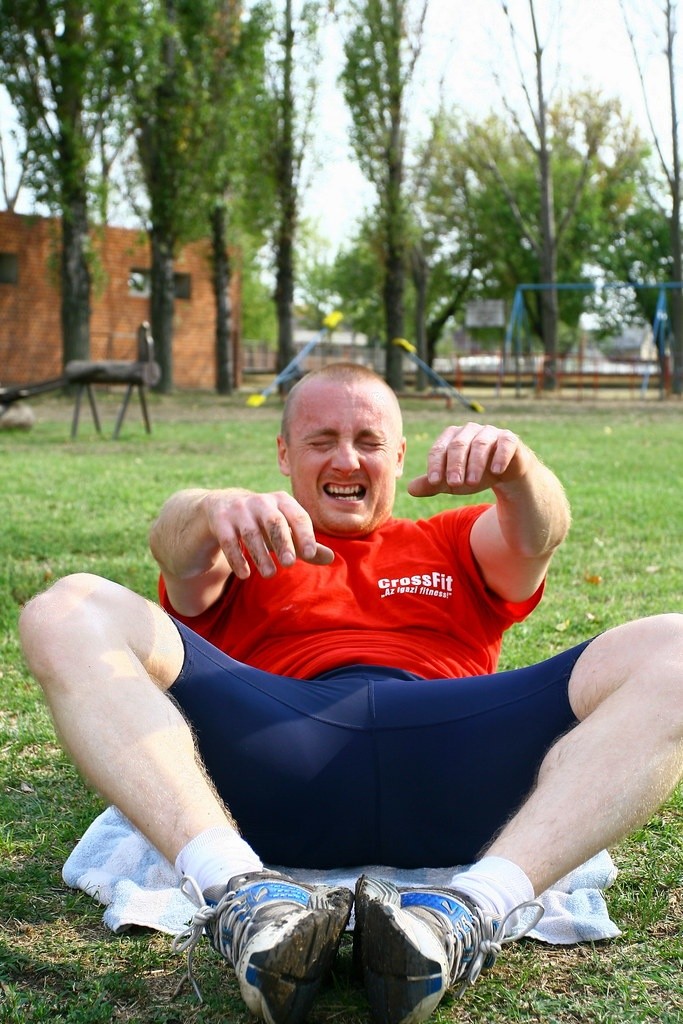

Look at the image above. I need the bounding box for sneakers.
[172,868,355,1024]
[355,874,545,1024]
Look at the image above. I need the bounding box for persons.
[20,363,683,1024]
